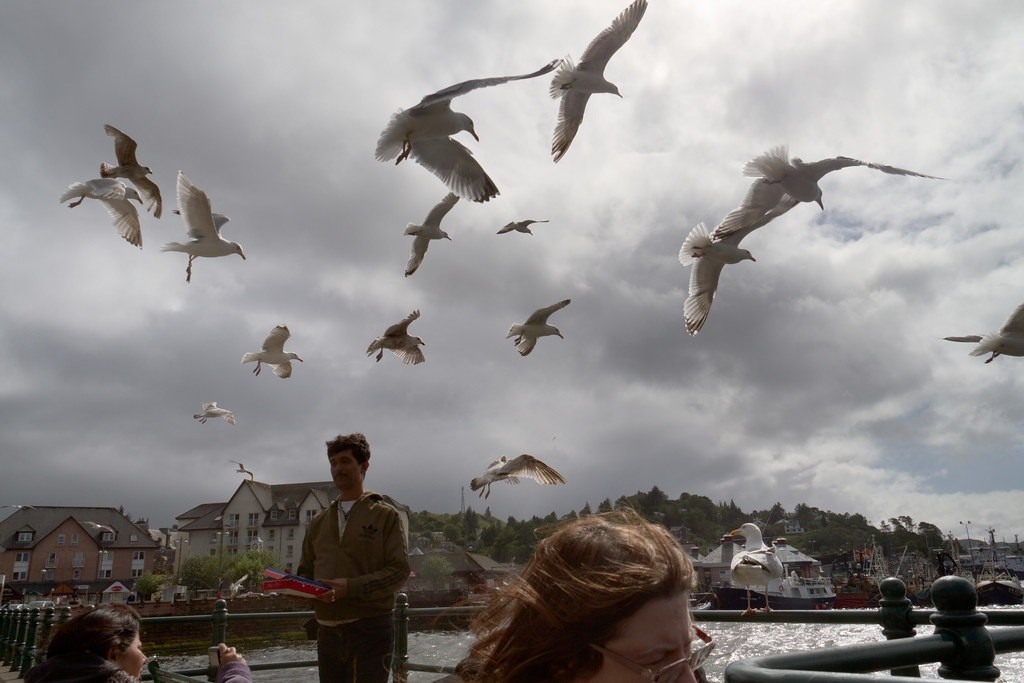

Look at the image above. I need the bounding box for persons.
[23,603,253,683]
[434,510,716,683]
[286,434,411,683]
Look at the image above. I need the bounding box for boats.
[711,526,1024,610]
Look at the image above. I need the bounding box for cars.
[1,600,54,622]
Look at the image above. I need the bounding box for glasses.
[584,625,716,683]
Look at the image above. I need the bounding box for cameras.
[208,646,222,667]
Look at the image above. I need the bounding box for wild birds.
[939,303,1023,364]
[497,218,550,235]
[507,298,572,356]
[373,59,565,204]
[366,309,426,365]
[79,521,115,534]
[678,141,949,338]
[0,504,39,511]
[59,178,143,251]
[549,0,649,164]
[194,401,237,426]
[229,574,248,603]
[160,171,246,283]
[403,191,460,276]
[731,524,784,612]
[242,324,303,379]
[469,453,567,500]
[100,124,162,219]
[230,460,253,482]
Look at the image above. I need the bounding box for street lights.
[175,536,188,585]
[216,532,229,597]
[959,521,973,563]
[99,546,107,578]
